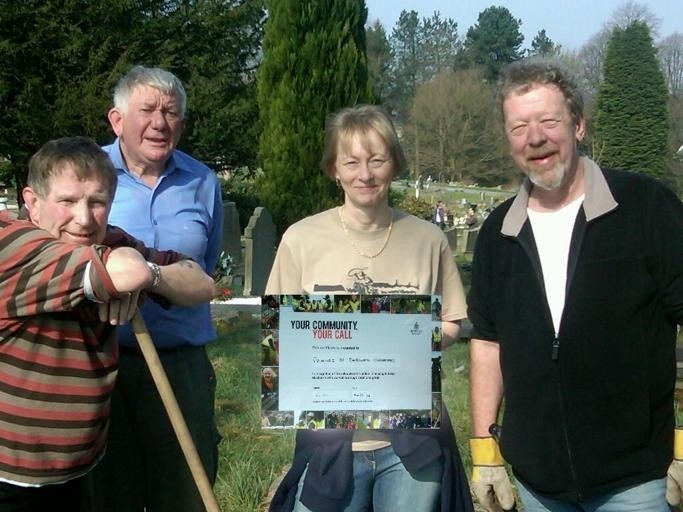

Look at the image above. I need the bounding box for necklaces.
[339,201,395,259]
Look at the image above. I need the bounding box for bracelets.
[146,260,161,292]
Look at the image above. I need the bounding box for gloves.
[666,425,683,512]
[468,435,515,512]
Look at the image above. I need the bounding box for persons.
[415,174,502,232]
[95,64,225,511]
[432,314,441,426]
[463,60,683,511]
[261,410,433,429]
[1,135,217,512]
[279,295,442,315]
[261,293,279,411]
[259,104,479,511]
